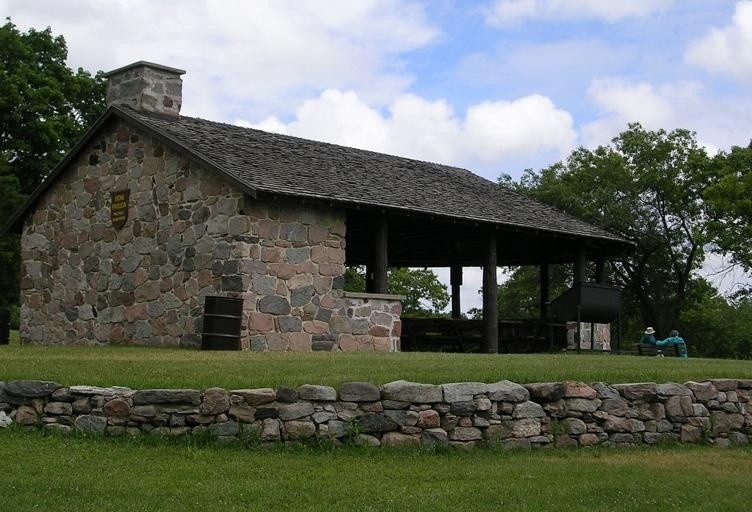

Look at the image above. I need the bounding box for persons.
[640,326,656,345]
[656,329,687,358]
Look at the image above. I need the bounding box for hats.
[644,327,656,335]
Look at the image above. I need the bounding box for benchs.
[631,342,686,357]
